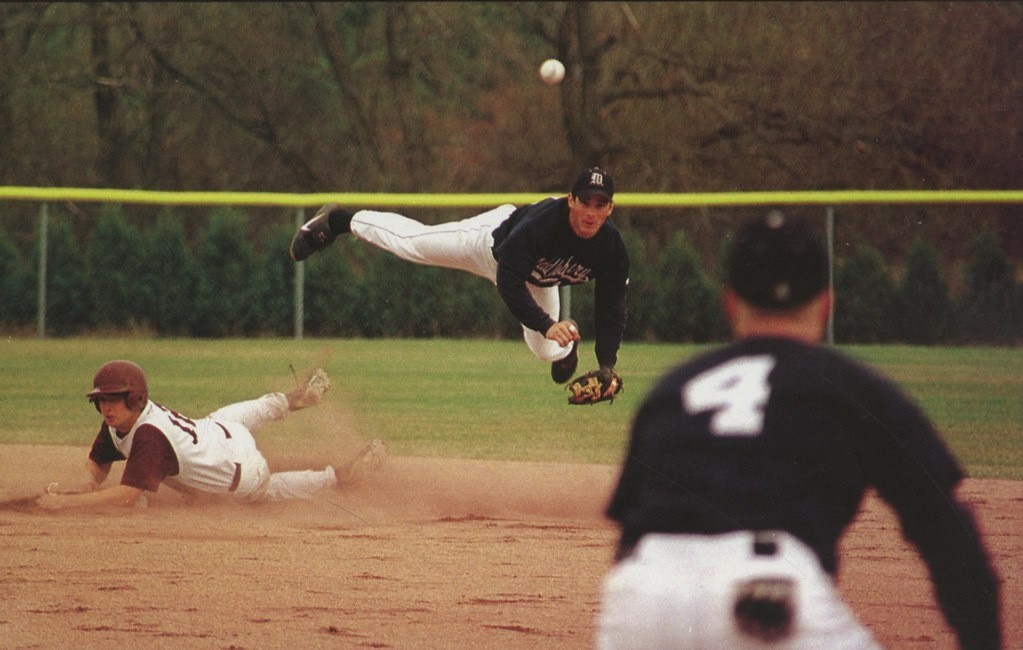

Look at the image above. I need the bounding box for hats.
[571,166,615,206]
[718,209,832,312]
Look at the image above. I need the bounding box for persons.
[288,167,628,408]
[0,356,384,512]
[592,215,1005,650]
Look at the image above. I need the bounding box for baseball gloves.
[564,366,625,406]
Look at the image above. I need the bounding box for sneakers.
[550,318,580,384]
[289,201,349,263]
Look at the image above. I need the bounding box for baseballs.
[539,58,566,85]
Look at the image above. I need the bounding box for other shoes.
[335,438,386,495]
[286,367,331,412]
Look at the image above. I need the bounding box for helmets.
[88,358,150,415]
